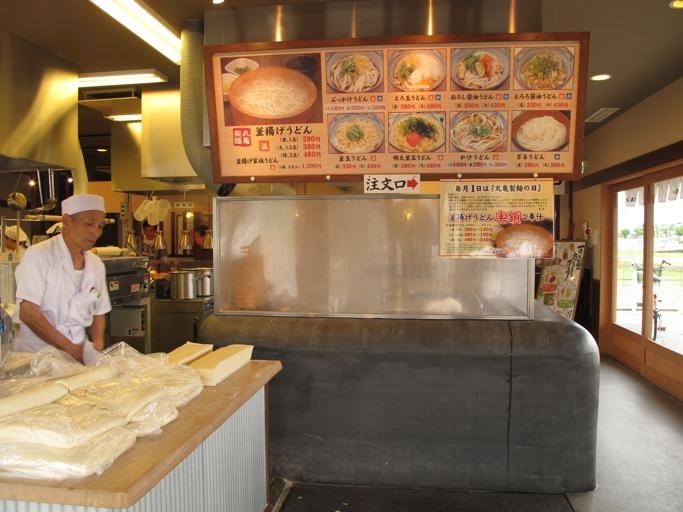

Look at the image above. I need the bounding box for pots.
[8,192,26,254]
[169,271,212,300]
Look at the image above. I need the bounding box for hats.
[61,194,106,217]
[6,226,26,242]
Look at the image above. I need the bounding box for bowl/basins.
[495,221,554,257]
[221,56,321,125]
[326,46,577,152]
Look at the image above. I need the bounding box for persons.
[15,192,113,366]
[4,225,28,255]
[143,220,160,257]
[195,226,209,255]
